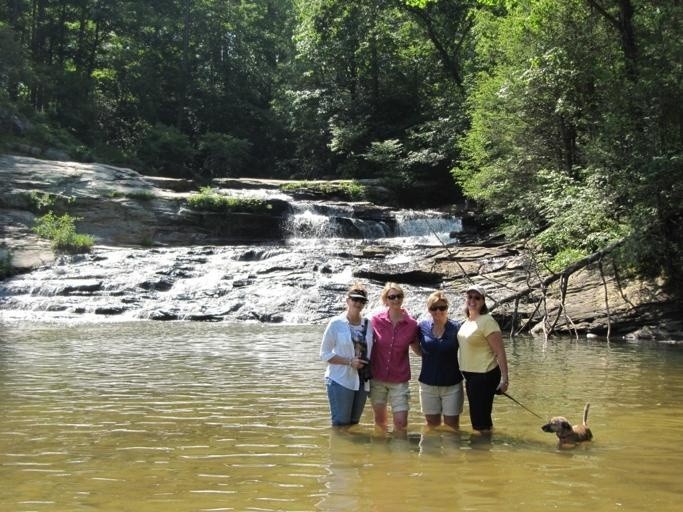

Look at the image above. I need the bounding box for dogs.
[540,402,593,449]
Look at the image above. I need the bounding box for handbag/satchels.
[356,357,373,382]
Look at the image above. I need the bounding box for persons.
[318,287,374,426]
[368,281,422,428]
[455,284,510,432]
[414,291,462,427]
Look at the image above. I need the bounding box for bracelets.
[500,380,508,386]
[348,359,352,366]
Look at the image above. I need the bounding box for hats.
[347,286,370,302]
[466,284,485,296]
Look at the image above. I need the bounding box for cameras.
[360,357,371,382]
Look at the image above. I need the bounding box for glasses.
[466,295,483,300]
[386,293,404,300]
[429,304,448,311]
[350,298,367,304]
